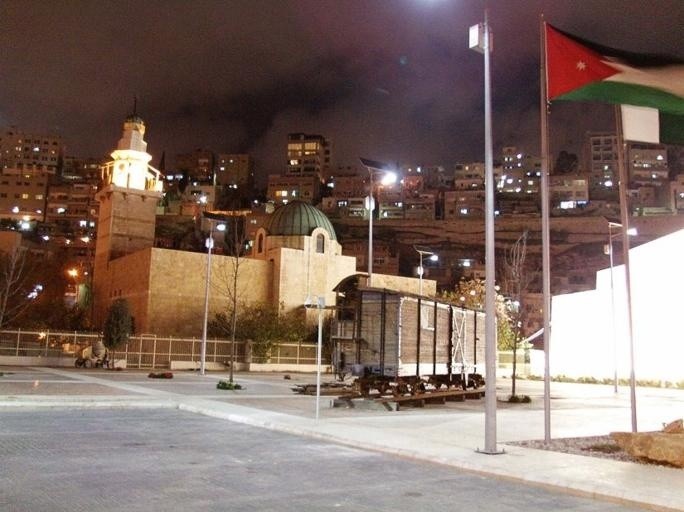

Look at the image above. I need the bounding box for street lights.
[627,228,638,236]
[201,236,215,250]
[416,267,424,277]
[430,254,438,261]
[380,172,398,186]
[216,223,226,231]
[604,244,614,257]
[507,298,522,308]
[462,262,470,267]
[305,295,325,311]
[362,195,377,210]
